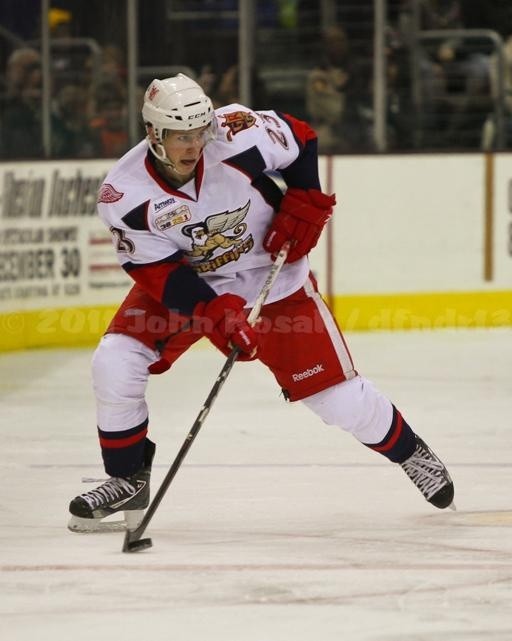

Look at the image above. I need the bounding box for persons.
[69,72,452,519]
[308,22,493,154]
[4,10,270,158]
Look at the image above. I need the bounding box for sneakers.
[70,468,149,519]
[399,432,453,509]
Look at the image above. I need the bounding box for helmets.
[142,73,219,168]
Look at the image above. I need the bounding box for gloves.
[262,190,336,266]
[191,292,258,360]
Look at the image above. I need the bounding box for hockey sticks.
[122,238,291,553]
